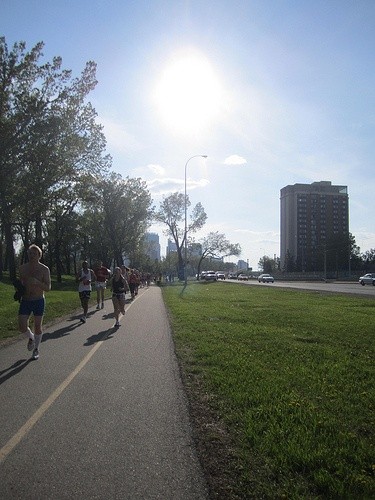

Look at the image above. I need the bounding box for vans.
[205,270,216,281]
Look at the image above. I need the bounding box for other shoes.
[96,303,104,310]
[122,310,125,315]
[33,349,40,360]
[79,317,86,323]
[27,338,34,352]
[115,321,120,327]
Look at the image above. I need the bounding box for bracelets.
[78,278,81,280]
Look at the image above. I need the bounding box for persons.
[76,261,97,322]
[94,261,150,325]
[14,244,52,360]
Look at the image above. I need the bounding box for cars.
[257,274,274,284]
[201,270,206,279]
[237,274,248,280]
[228,272,236,279]
[358,273,375,286]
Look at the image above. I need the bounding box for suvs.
[216,271,225,280]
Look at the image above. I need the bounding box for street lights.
[184,154,208,288]
